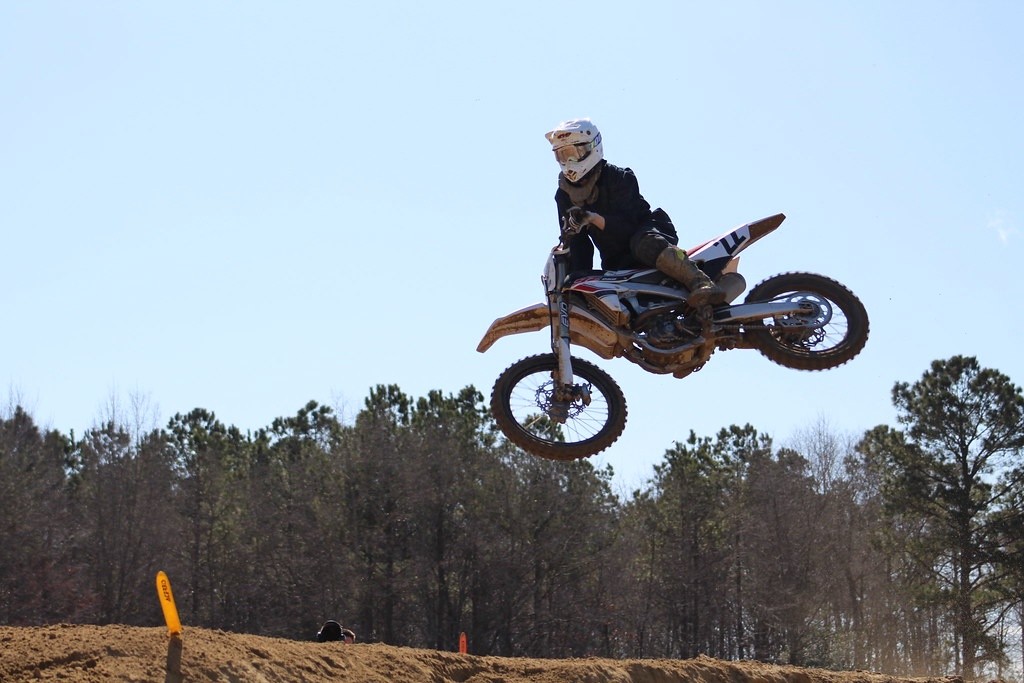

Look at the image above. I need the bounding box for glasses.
[552,142,591,167]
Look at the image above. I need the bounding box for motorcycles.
[477,208,872,462]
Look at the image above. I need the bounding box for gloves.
[560,206,598,235]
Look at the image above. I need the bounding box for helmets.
[545,121,603,184]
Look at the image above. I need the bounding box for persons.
[544,117,727,379]
[315,620,356,645]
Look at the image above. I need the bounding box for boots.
[649,245,728,307]
